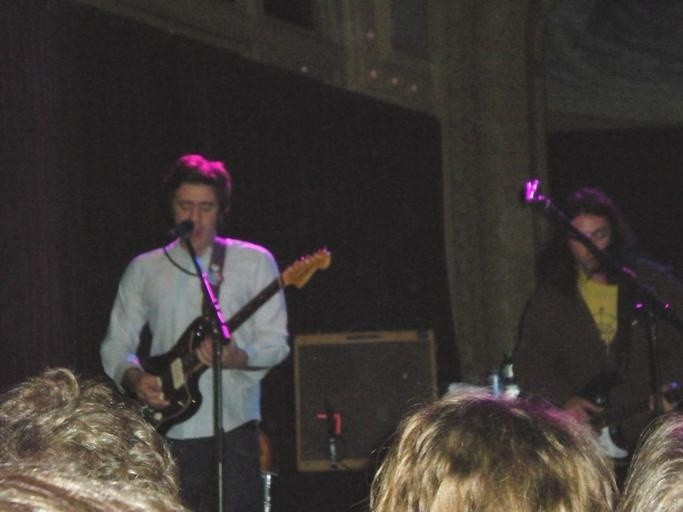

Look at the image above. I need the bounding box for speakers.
[272,328,439,473]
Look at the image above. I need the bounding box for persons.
[99,154,291,512]
[366,383,620,511]
[511,186,683,456]
[0,365,194,512]
[615,407,683,512]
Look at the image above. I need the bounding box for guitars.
[137,247,332,438]
[578,372,683,458]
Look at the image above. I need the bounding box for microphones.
[163,220,194,246]
[325,400,338,468]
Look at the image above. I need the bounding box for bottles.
[487,354,518,403]
